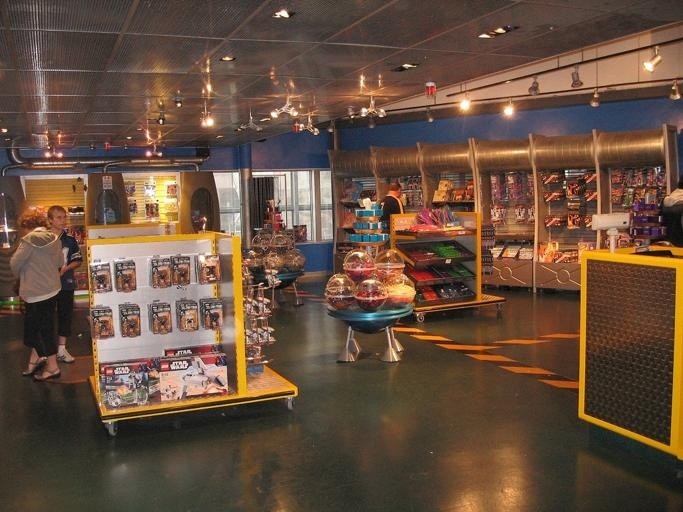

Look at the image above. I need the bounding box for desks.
[251,269,304,309]
[326,301,414,362]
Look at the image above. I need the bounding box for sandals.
[33,364,60,382]
[21,356,46,376]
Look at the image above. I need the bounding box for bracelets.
[69,265,71,270]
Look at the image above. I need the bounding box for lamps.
[570,64,583,88]
[528,75,540,96]
[643,46,662,73]
[669,80,681,100]
[589,88,600,108]
[357,96,387,118]
[270,96,297,118]
[237,106,263,132]
[297,113,320,136]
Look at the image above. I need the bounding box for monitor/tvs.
[630,249,675,258]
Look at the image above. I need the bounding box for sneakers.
[56,348,75,363]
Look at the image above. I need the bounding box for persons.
[374,181,405,229]
[661,175,683,248]
[44,206,83,365]
[8,205,64,380]
